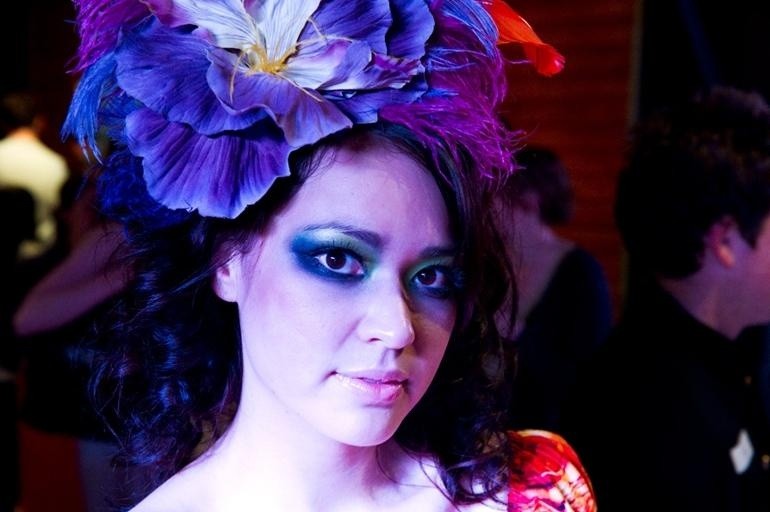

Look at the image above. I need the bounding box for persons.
[479,85,770,511]
[0,86,181,512]
[70,0,600,512]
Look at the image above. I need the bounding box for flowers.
[109,0,438,223]
